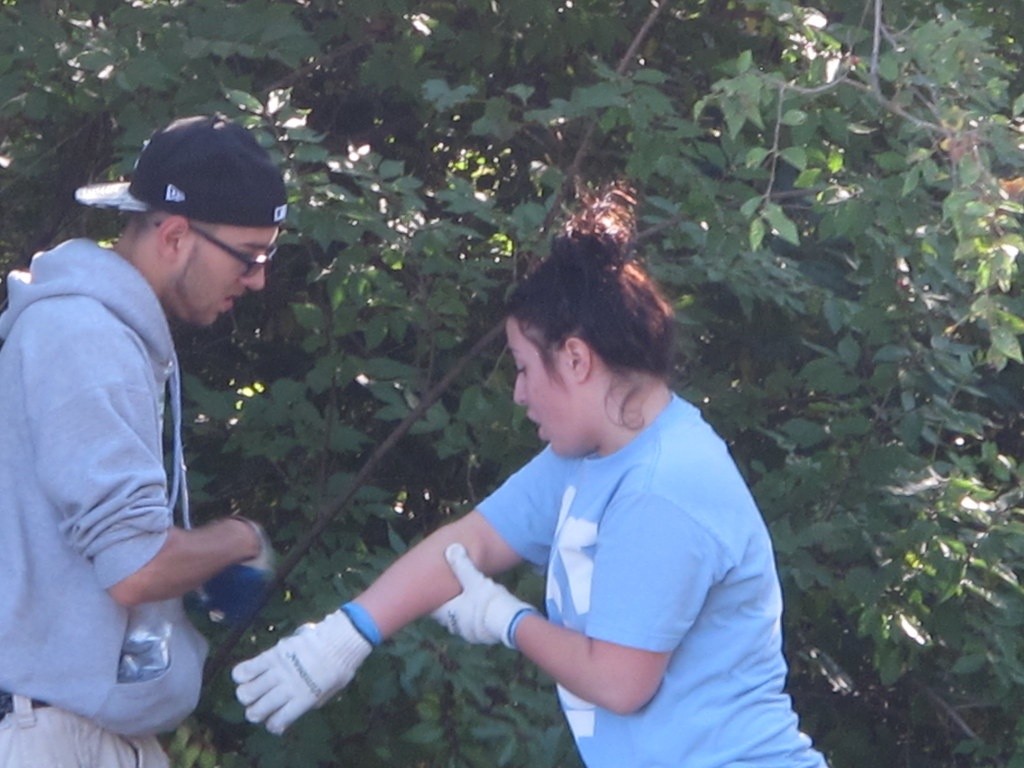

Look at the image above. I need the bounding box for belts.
[0,693,49,715]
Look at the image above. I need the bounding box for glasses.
[153,220,273,272]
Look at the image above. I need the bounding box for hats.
[75,116,287,227]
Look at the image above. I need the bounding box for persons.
[0,112,289,766]
[231,203,828,768]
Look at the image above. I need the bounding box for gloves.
[428,543,538,650]
[193,515,275,626]
[232,601,382,735]
